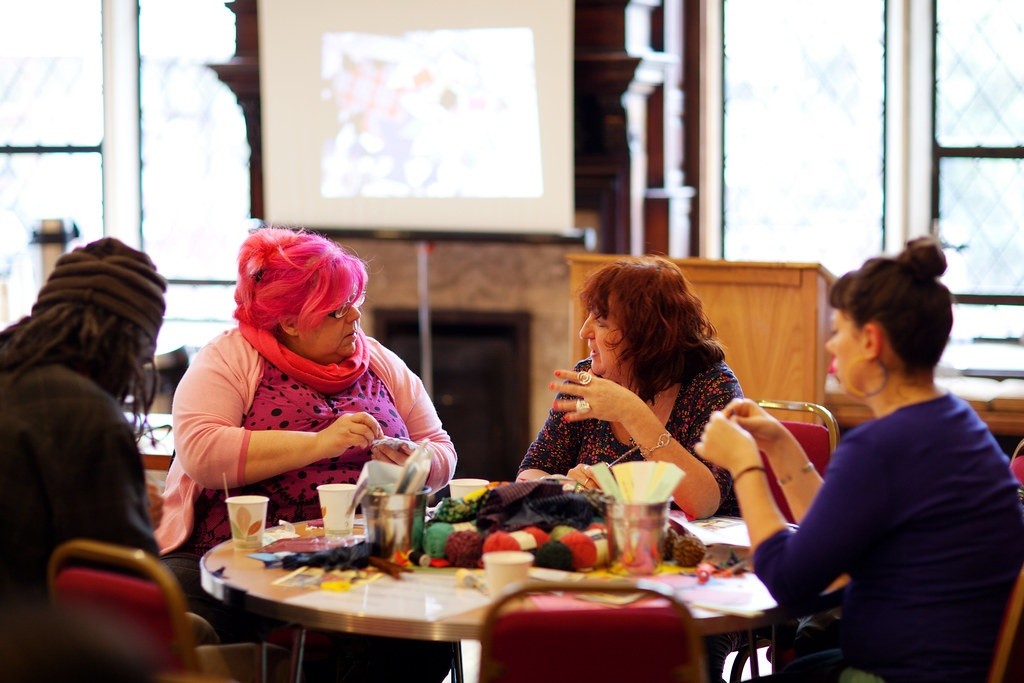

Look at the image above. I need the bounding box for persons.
[0,227,458,683]
[696,236,1024,683]
[515,252,766,683]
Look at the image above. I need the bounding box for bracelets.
[777,461,814,486]
[730,466,765,482]
[641,430,672,457]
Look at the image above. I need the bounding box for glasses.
[328,289,366,319]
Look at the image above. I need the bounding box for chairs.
[51,400,1024,683]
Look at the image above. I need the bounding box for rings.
[577,371,592,385]
[576,399,591,414]
[584,477,591,486]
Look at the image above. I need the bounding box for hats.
[32,236,168,346]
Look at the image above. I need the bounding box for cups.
[362,484,432,563]
[225,496,270,551]
[600,495,675,576]
[482,552,535,595]
[448,479,489,501]
[317,483,358,539]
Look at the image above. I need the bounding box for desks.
[197,504,852,682]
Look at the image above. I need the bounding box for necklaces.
[629,397,659,441]
